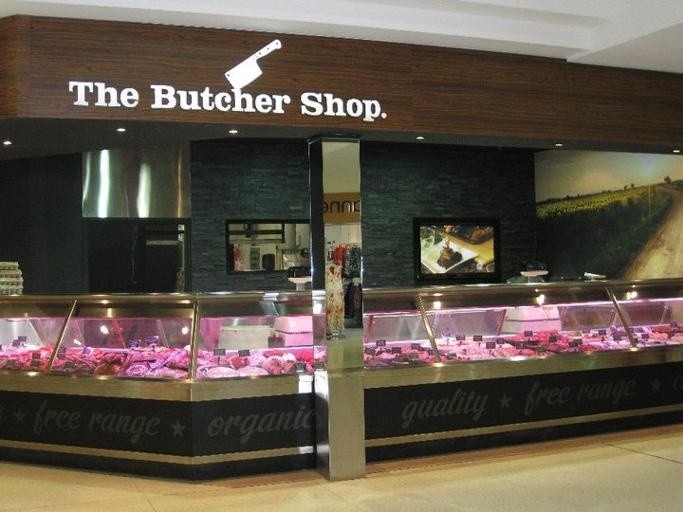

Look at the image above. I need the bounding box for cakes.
[437,246,462,269]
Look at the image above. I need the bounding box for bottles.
[326,238,337,261]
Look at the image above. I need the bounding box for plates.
[421,235,478,274]
